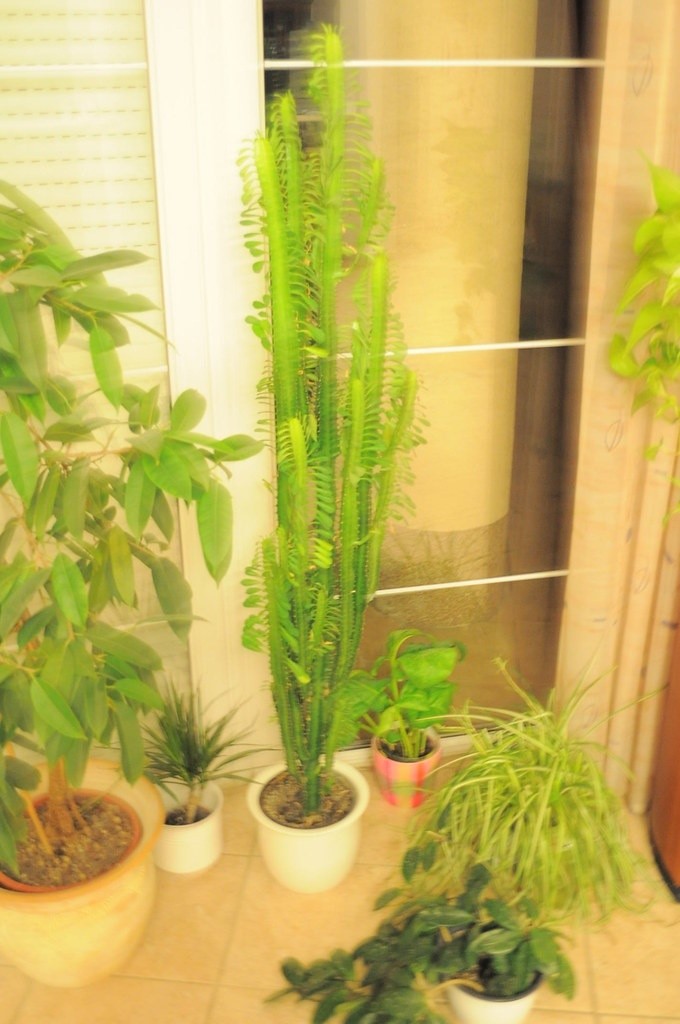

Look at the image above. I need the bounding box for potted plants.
[352,630,464,806]
[406,661,671,934]
[1,176,264,990]
[265,843,576,1024]
[96,676,283,877]
[235,25,429,895]
[608,162,680,899]
